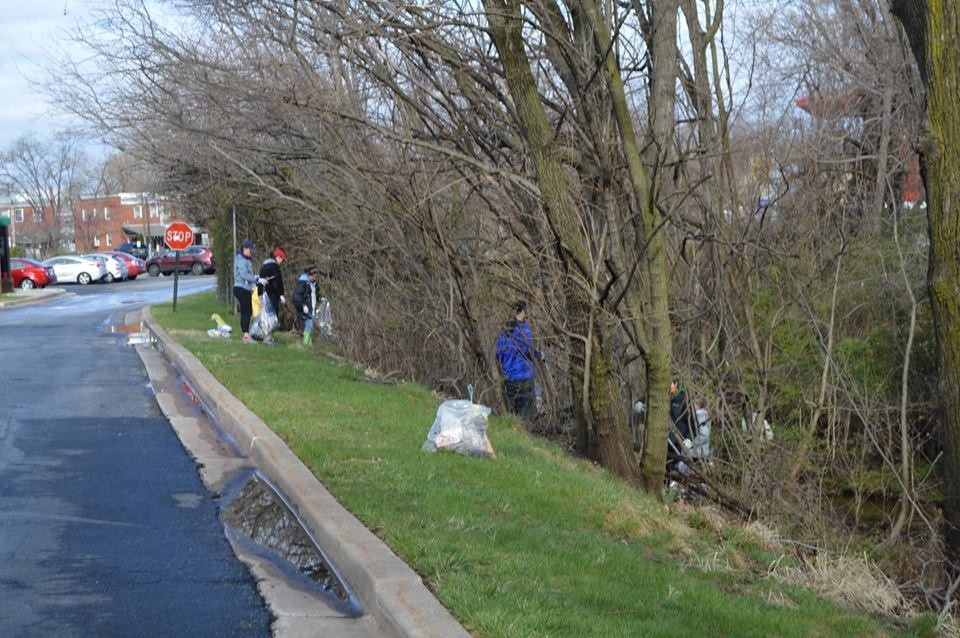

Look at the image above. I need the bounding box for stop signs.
[164,221,194,251]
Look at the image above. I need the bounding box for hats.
[309,266,318,274]
[272,249,288,262]
[241,240,256,248]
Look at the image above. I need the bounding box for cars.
[41,254,109,285]
[80,255,129,283]
[95,251,146,281]
[5,257,57,290]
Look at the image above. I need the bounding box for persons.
[633,378,773,493]
[292,265,328,347]
[232,240,268,343]
[257,248,286,344]
[495,300,545,422]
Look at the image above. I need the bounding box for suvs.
[146,245,216,277]
[115,244,146,259]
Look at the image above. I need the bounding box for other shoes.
[241,333,313,349]
